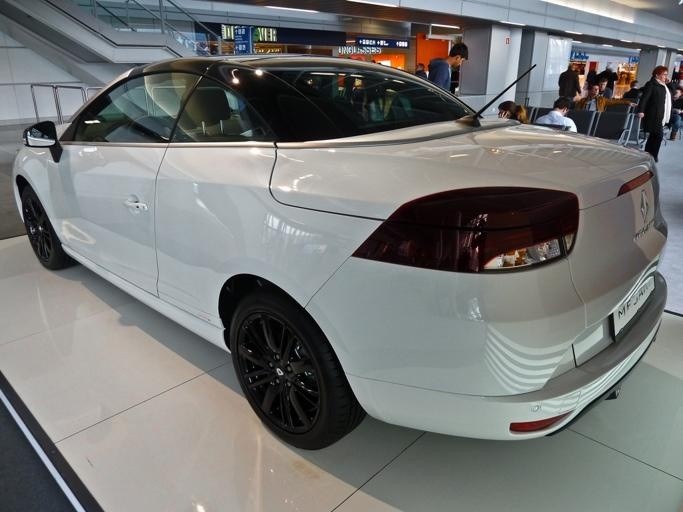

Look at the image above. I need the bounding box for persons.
[558,57,638,112]
[498,101,525,123]
[427,42,469,93]
[638,66,671,163]
[670,89,683,139]
[413,63,425,78]
[535,98,577,134]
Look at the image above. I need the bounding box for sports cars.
[10,55,669,449]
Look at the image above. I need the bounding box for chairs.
[173,87,231,137]
[513,92,682,150]
